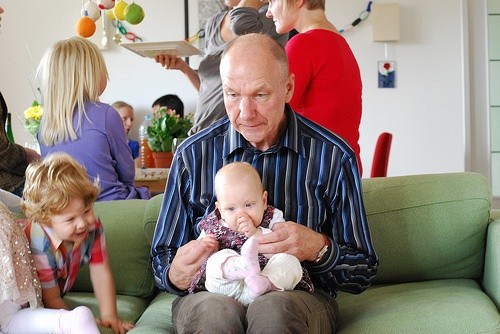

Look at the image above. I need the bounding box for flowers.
[146,106,194,152]
[22,101,44,136]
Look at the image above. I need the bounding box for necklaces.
[0,138,11,155]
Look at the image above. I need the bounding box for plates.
[118,40,206,58]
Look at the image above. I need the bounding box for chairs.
[370,132,392,178]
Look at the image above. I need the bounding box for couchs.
[64,171,500,334]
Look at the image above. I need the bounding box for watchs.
[314,233,329,262]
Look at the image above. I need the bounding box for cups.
[171,137,184,155]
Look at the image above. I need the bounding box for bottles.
[7,113,14,144]
[138,115,155,168]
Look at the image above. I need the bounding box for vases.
[152,151,173,167]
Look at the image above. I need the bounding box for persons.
[15,153,135,334]
[152,95,184,118]
[151,33,380,334]
[266,0,363,179]
[0,92,40,196]
[36,38,151,202]
[0,202,100,334]
[187,163,313,306]
[110,101,141,168]
[157,0,290,134]
